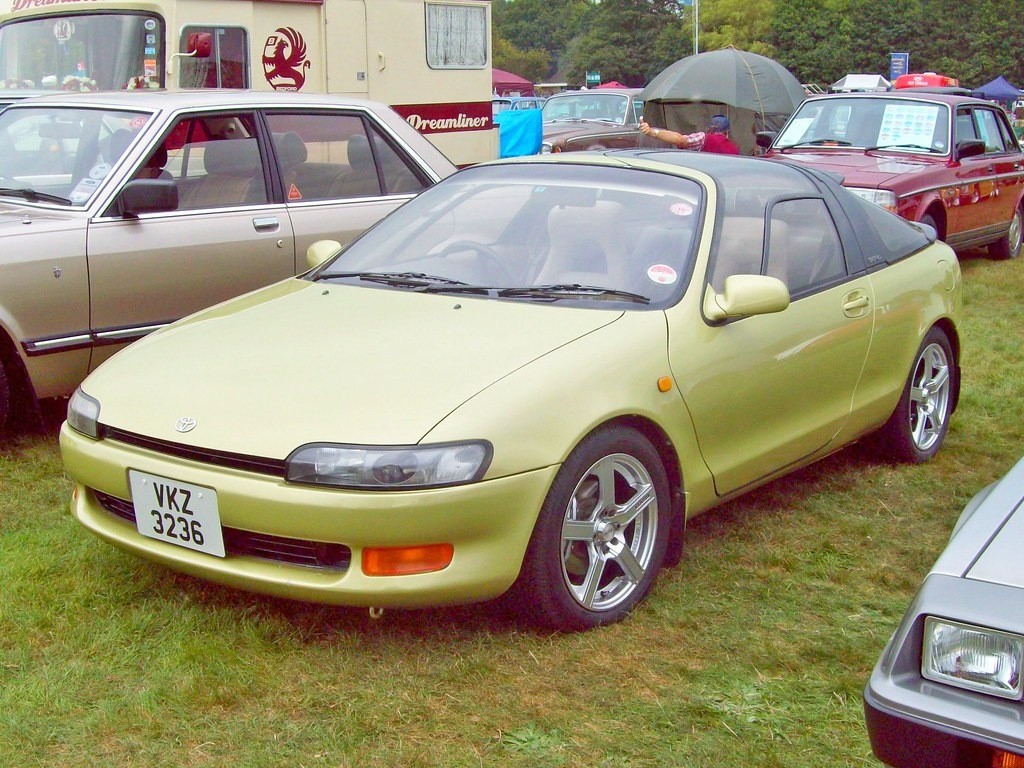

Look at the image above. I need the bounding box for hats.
[707,115,730,131]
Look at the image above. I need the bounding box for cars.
[537,88,648,156]
[56,146,965,634]
[1,87,624,430]
[753,90,1024,260]
[861,453,1024,768]
[491,93,548,121]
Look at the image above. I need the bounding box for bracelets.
[654,129,658,138]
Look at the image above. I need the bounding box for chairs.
[703,216,787,297]
[189,139,261,207]
[104,129,176,184]
[538,202,630,292]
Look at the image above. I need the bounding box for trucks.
[1,0,501,188]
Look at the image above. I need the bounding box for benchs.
[270,130,407,200]
[621,206,836,290]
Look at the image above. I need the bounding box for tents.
[597,80,629,89]
[970,75,1024,111]
[492,67,535,98]
[638,44,809,157]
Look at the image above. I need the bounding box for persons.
[639,115,740,155]
